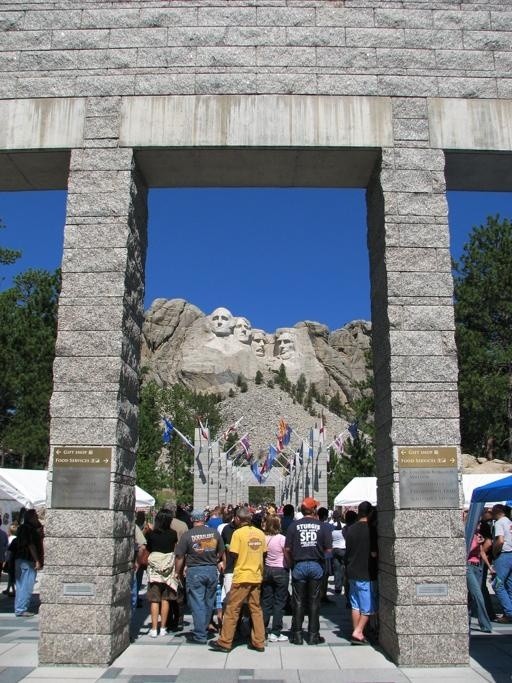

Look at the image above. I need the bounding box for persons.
[462,504,512,633]
[0,507,42,616]
[130,496,377,652]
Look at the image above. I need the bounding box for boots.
[308,631,326,646]
[292,631,304,646]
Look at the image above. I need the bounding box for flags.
[160,413,360,483]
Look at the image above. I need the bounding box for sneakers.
[148,627,159,637]
[268,632,290,644]
[160,627,170,636]
[246,641,265,653]
[492,616,512,624]
[209,639,232,655]
[21,611,35,618]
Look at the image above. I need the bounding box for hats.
[301,495,321,511]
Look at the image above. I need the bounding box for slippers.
[350,636,371,646]
